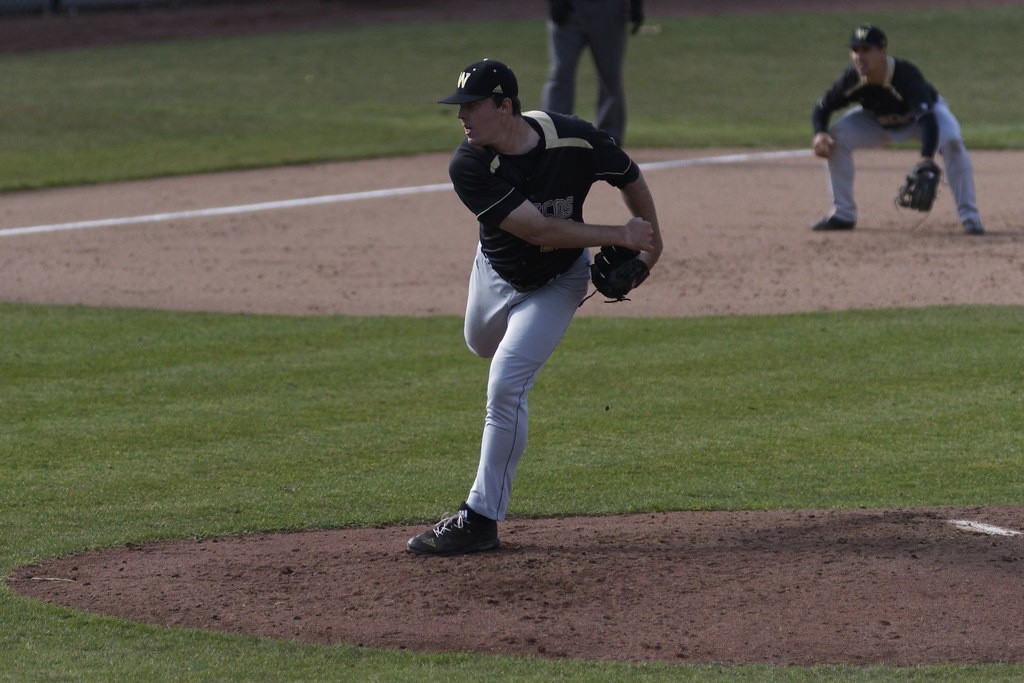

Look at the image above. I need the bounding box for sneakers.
[812,215,856,230]
[961,218,985,233]
[406,500,501,555]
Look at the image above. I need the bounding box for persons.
[543,0,646,143]
[811,23,988,235]
[406,58,663,555]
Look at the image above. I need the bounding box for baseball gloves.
[897,161,941,215]
[590,241,648,300]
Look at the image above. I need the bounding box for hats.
[436,58,519,105]
[845,24,888,48]
